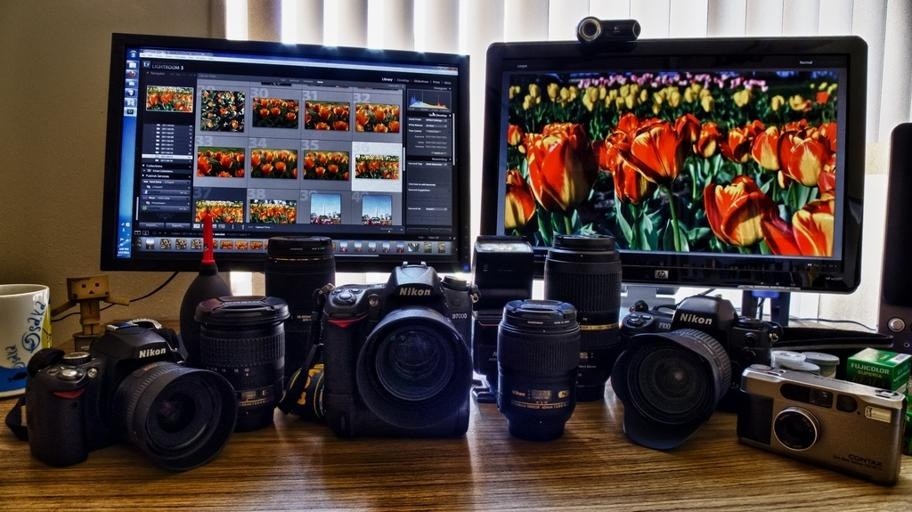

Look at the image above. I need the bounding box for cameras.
[611,297,777,450]
[319,261,470,442]
[740,369,904,489]
[27,319,237,474]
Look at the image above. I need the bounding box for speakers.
[881,122,912,352]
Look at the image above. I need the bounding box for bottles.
[772,350,840,379]
[179,205,234,356]
[898,366,912,457]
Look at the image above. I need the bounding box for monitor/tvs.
[482,36,867,292]
[100,29,471,274]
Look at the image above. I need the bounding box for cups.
[0,284,51,402]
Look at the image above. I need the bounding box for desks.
[2,316,912,512]
